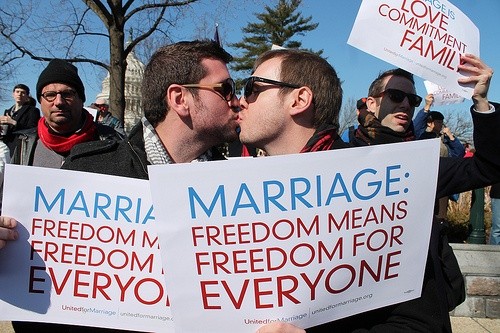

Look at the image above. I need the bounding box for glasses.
[41,89,79,102]
[244,76,302,97]
[374,88,423,107]
[162,78,236,101]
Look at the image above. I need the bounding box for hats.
[95,97,109,105]
[356,97,368,108]
[36,58,86,104]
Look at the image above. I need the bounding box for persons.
[0,84,40,138]
[10,58,123,168]
[348,53,500,219]
[0,40,238,333]
[238,50,465,333]
[341,92,499,245]
[88,97,122,130]
